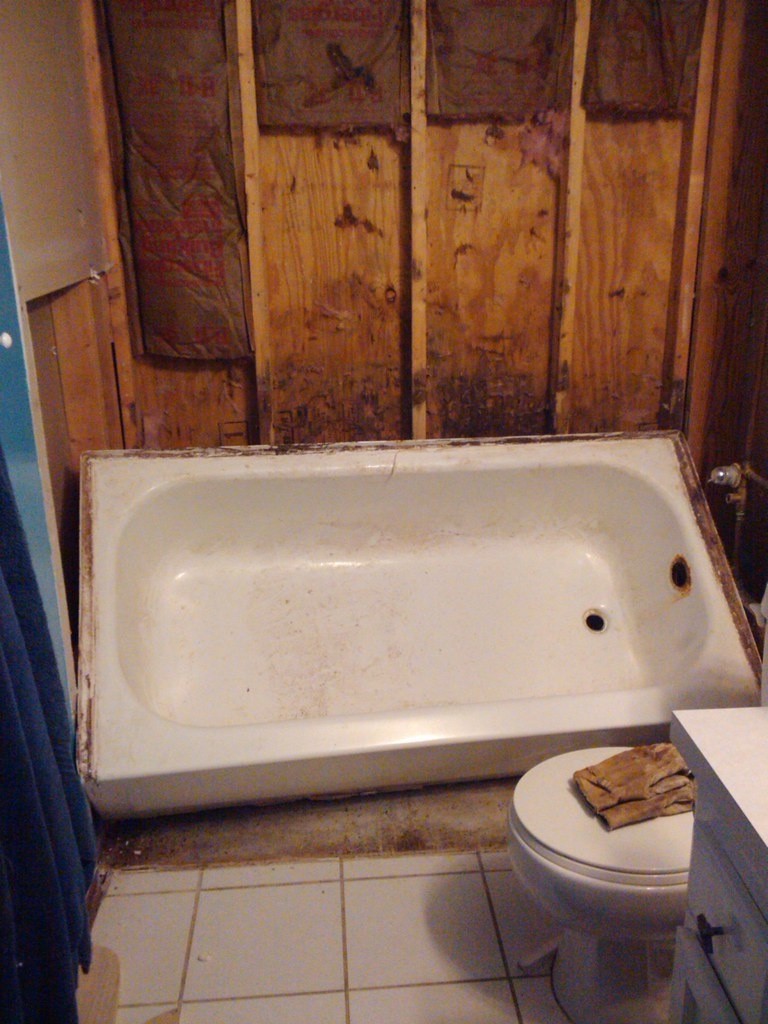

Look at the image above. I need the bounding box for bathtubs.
[73,429,762,819]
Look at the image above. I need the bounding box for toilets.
[503,744,695,1000]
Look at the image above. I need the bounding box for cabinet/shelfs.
[662,804,768,1024]
